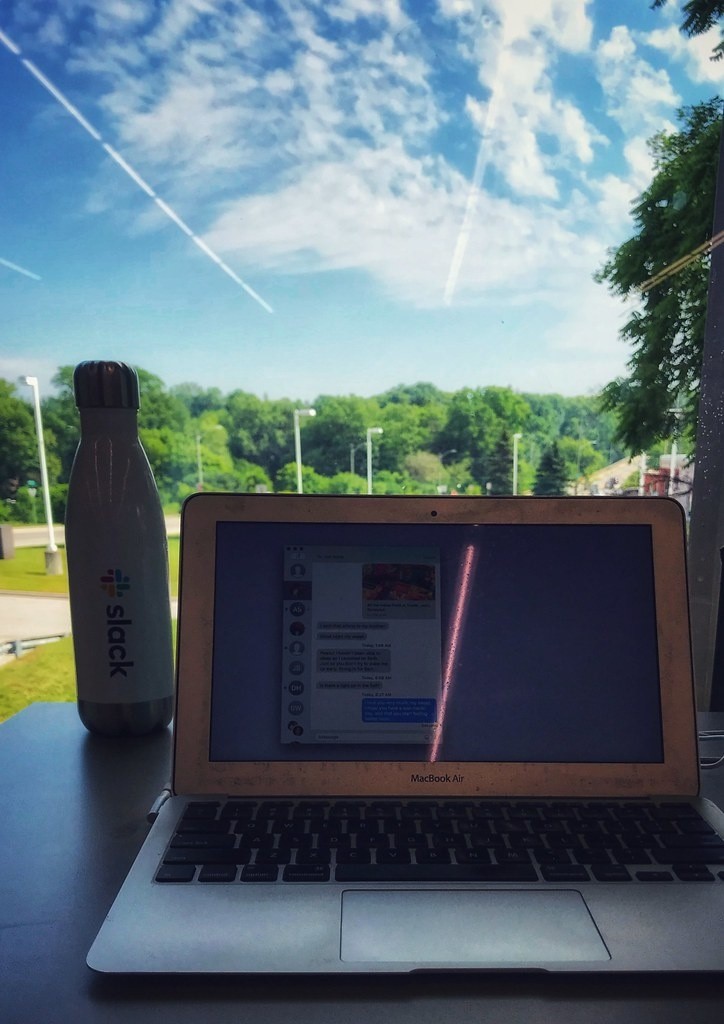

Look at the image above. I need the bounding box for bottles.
[62,359,175,737]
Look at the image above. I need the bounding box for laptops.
[85,489,724,983]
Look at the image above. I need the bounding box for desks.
[0,702,724,1024]
